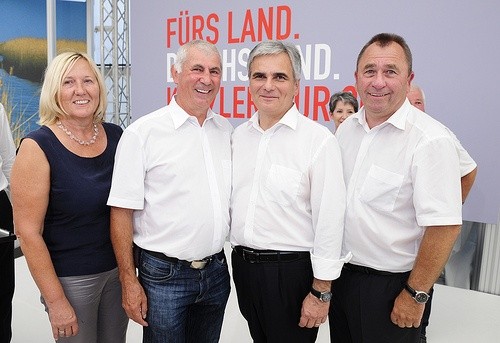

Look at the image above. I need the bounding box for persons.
[408,87,478,284]
[329,92,358,129]
[12,52,136,343]
[228,40,353,343]
[105,39,234,343]
[327,33,462,343]
[0,103,17,343]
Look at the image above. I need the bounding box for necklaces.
[57,121,98,145]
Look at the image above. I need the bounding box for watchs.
[404,283,430,304]
[310,287,332,303]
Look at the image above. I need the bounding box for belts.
[234,246,310,263]
[143,248,224,270]
